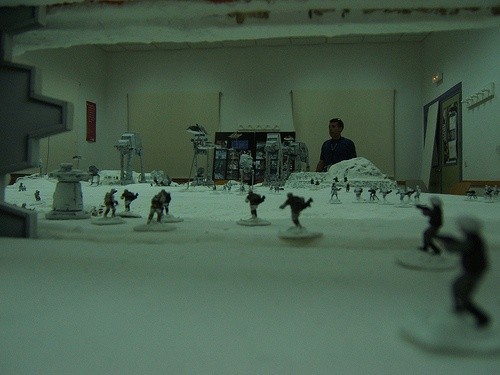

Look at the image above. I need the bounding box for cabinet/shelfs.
[215,129,297,183]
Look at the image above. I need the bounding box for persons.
[420,198,443,256]
[465,184,500,202]
[244,190,259,218]
[381,185,421,204]
[310,178,320,191]
[279,193,304,228]
[446,215,492,327]
[101,189,118,219]
[120,189,133,212]
[316,118,357,171]
[159,189,170,214]
[147,196,163,225]
[354,182,363,202]
[330,174,350,200]
[368,182,378,201]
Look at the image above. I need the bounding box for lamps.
[431,70,445,83]
